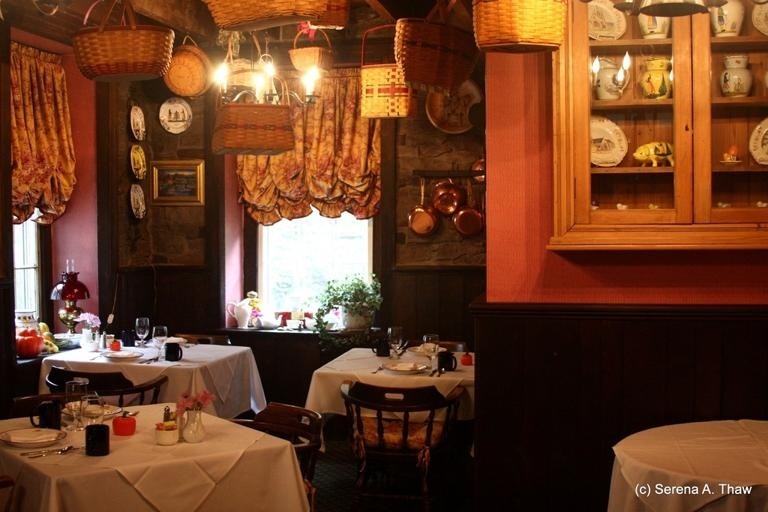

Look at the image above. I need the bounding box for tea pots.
[254,307,284,330]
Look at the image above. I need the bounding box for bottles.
[632,1,676,98]
[708,1,768,99]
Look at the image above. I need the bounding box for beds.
[311,345,476,486]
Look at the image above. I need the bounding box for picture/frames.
[143,157,206,207]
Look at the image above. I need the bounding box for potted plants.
[311,273,387,359]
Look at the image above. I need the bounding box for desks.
[213,324,381,407]
[606,420,767,511]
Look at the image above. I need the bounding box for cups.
[111,341,120,350]
[165,343,183,361]
[113,415,137,437]
[285,316,338,332]
[84,424,110,457]
[27,399,62,432]
[369,326,458,374]
[461,353,472,366]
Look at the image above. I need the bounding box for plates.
[157,97,194,135]
[0,427,68,448]
[588,1,632,167]
[105,349,145,361]
[61,402,124,420]
[421,79,482,137]
[128,105,149,222]
[748,115,768,168]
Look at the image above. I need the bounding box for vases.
[78,328,99,352]
[180,407,206,445]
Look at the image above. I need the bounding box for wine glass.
[134,317,166,366]
[63,379,103,431]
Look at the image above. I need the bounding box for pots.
[406,159,485,239]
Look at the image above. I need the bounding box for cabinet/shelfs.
[544,0,768,255]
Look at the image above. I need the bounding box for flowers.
[74,311,100,331]
[173,386,218,413]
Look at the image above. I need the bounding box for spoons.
[19,445,74,459]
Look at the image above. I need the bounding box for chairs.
[340,378,466,509]
[13,390,84,416]
[176,332,228,345]
[45,363,170,407]
[224,399,322,491]
[409,339,466,353]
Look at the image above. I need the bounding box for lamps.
[213,26,327,114]
[49,271,90,335]
